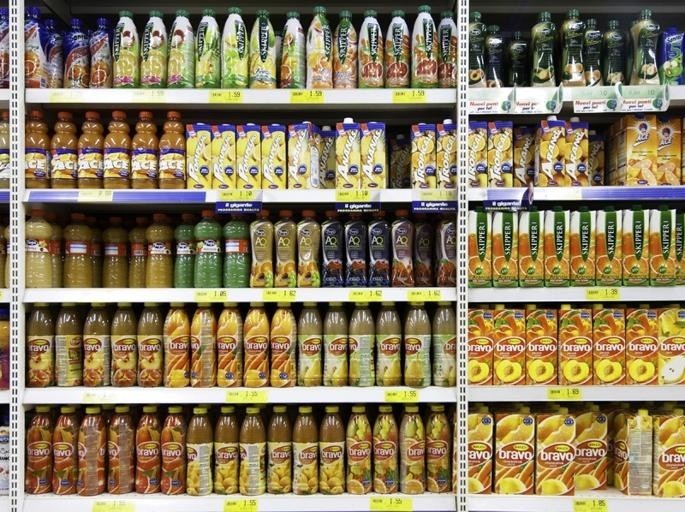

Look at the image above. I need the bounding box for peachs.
[468,401,685,500]
[469,304,685,385]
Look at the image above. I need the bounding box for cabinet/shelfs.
[9,2,465,512]
[457,0,685,510]
[0,1,11,512]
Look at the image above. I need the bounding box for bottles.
[1,4,685,88]
[0,111,185,188]
[2,203,457,288]
[1,404,453,496]
[1,302,456,389]
